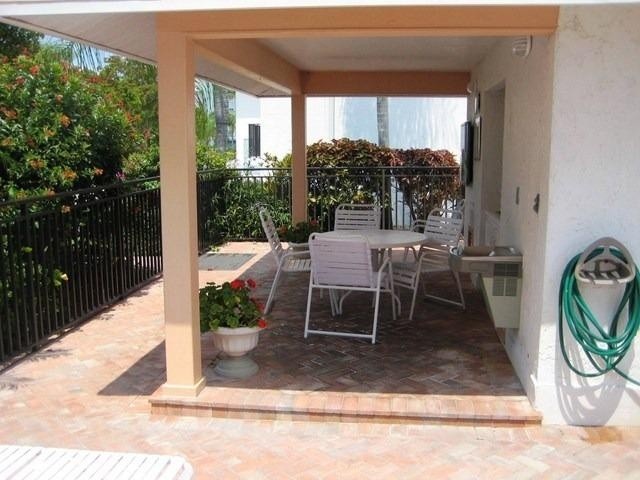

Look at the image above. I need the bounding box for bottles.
[456,236,465,256]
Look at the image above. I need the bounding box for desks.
[315,228,429,310]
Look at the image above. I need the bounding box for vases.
[210,328,260,382]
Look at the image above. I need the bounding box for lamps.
[467,80,476,96]
[512,36,532,57]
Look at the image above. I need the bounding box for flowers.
[196,280,265,327]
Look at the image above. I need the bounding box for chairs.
[256,205,334,318]
[336,200,383,234]
[304,231,397,343]
[397,210,471,317]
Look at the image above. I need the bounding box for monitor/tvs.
[460,121,473,186]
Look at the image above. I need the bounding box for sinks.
[448,245,524,279]
